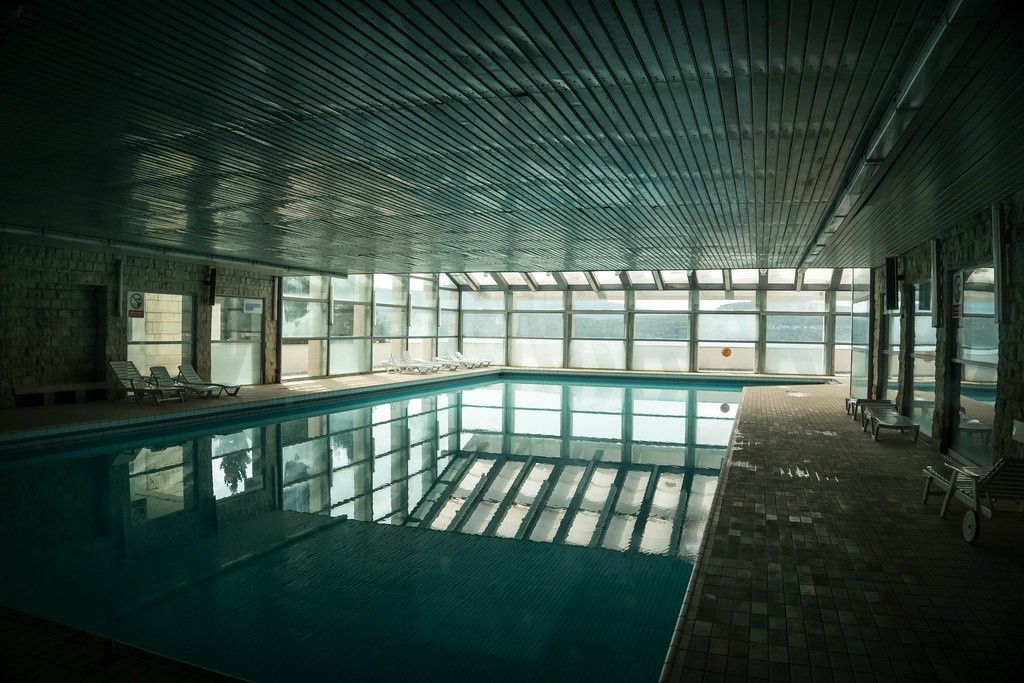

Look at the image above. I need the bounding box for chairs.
[844,397,920,442]
[380,351,494,375]
[109,360,246,406]
[921,456,1024,543]
